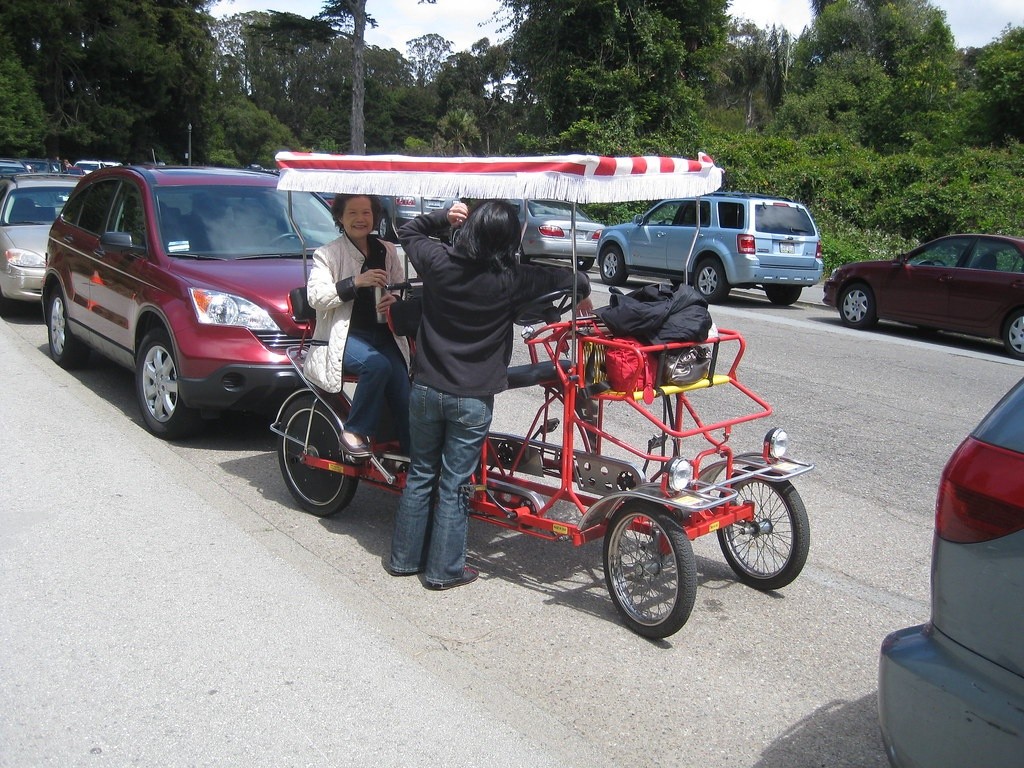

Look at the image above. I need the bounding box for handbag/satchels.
[605,337,658,404]
[662,346,712,386]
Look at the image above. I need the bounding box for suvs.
[595,190,824,308]
[38,162,343,441]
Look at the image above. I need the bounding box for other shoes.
[426,567,479,589]
[389,567,426,576]
[338,430,371,457]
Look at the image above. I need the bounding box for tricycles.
[267,151,812,639]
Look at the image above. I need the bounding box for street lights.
[187,123,193,167]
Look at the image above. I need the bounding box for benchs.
[386,298,578,390]
[287,285,425,384]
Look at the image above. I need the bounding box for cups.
[375,287,388,323]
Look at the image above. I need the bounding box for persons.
[391,200,593,591]
[303,194,410,466]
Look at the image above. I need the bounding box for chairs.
[979,253,997,271]
[12,198,34,217]
[229,203,289,249]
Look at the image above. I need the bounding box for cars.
[822,234,1024,361]
[378,195,463,243]
[876,372,1024,768]
[508,199,606,272]
[0,173,89,310]
[0,158,166,176]
